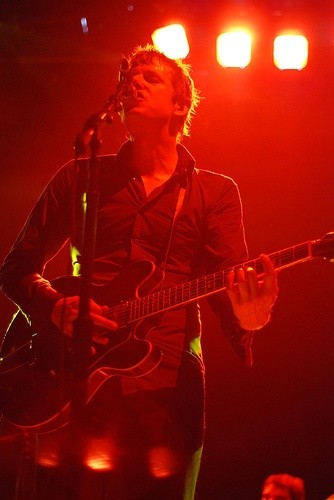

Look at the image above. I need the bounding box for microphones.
[119,56,137,100]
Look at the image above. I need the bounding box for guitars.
[0,228,334,435]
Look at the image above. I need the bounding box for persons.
[0,46,278,500]
